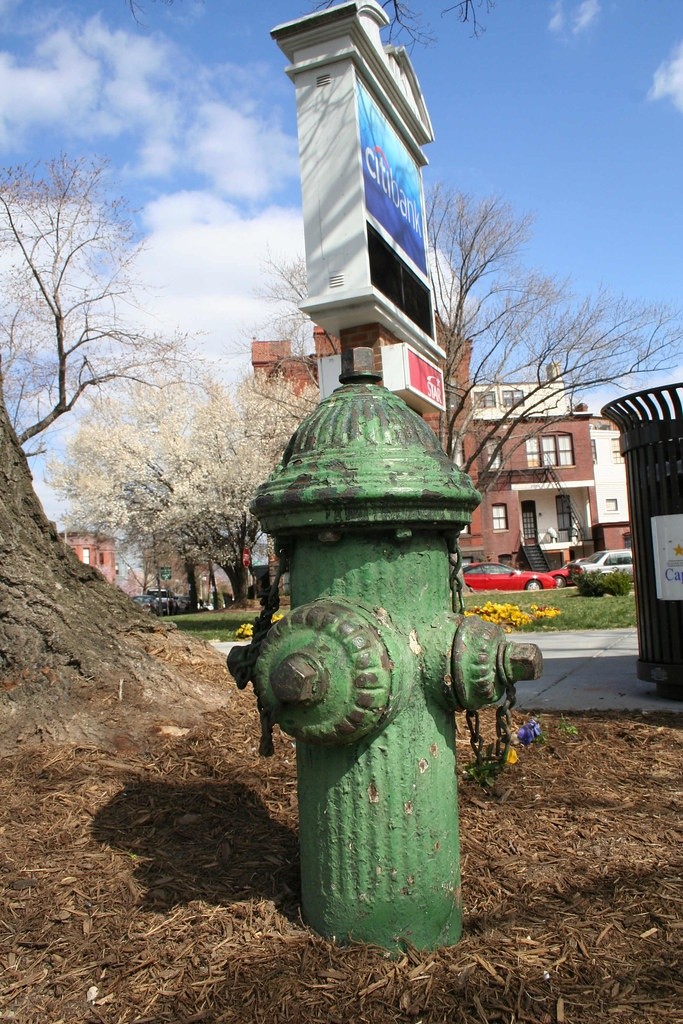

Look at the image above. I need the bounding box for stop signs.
[241,547,251,569]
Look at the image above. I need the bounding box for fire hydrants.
[223,347,546,960]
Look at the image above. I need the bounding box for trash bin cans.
[254,565,271,589]
[601,381,683,702]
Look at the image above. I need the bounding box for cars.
[460,561,558,593]
[567,549,635,584]
[129,588,235,617]
[545,557,589,587]
